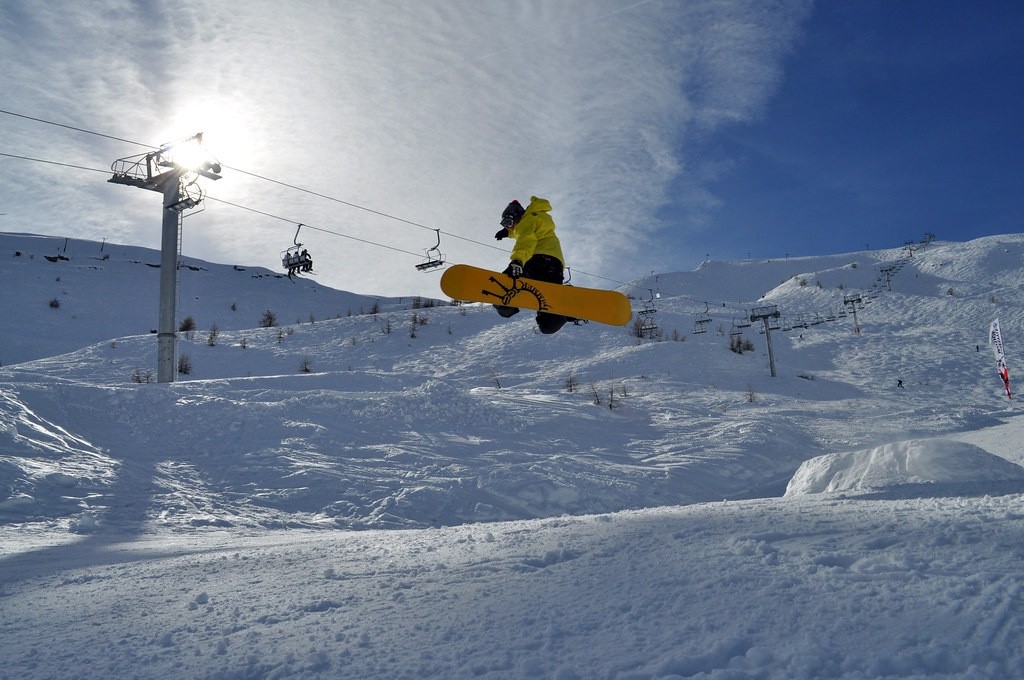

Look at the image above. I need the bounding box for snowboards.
[440,263,633,327]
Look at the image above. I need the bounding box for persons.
[492,196,589,334]
[287,249,313,276]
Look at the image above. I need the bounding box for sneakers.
[301,269,304,271]
[292,272,295,276]
[309,268,313,271]
[287,275,291,279]
[297,271,301,274]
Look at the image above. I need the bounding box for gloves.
[494,227,509,241]
[502,259,524,280]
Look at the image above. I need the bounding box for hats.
[287,252,290,256]
[304,249,307,252]
[502,200,525,218]
[295,252,298,254]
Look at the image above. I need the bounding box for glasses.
[500,220,513,227]
